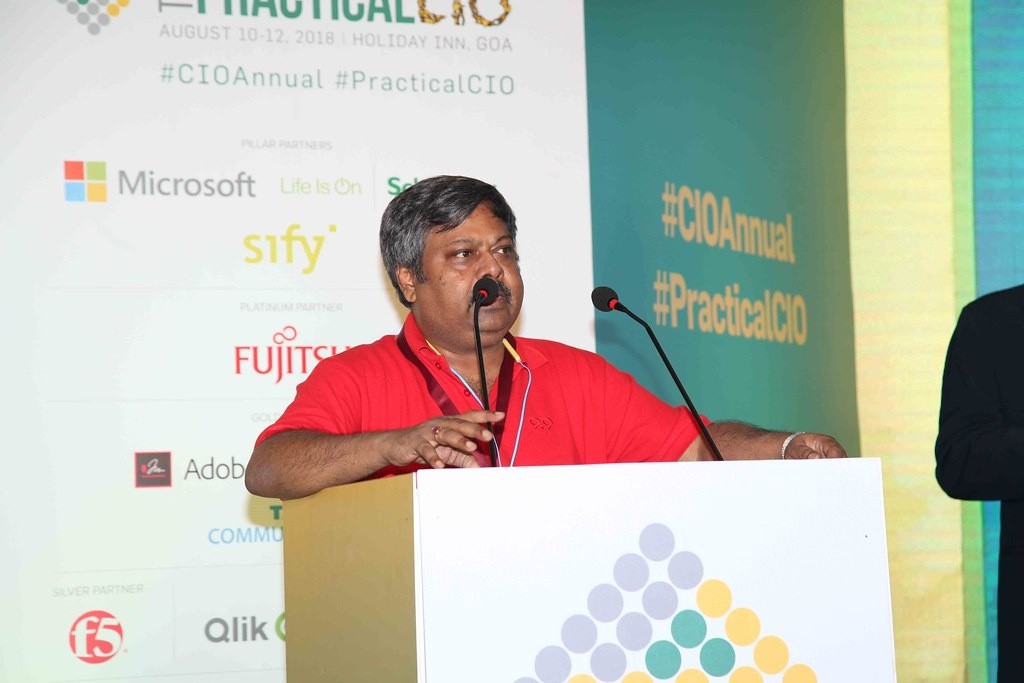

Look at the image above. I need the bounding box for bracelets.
[781,431,806,460]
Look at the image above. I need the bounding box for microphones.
[591,286,724,462]
[472,277,499,467]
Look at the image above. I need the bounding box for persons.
[245,174,846,501]
[934,284,1024,683]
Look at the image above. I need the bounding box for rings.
[430,425,447,446]
